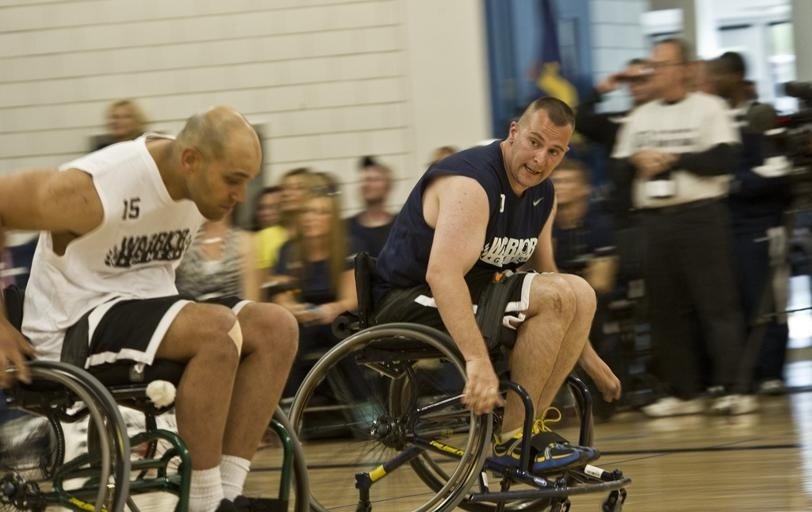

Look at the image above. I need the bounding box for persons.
[1,105,300,512]
[369,98,622,475]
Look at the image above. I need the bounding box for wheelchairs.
[287,254,631,511]
[1,283,309,512]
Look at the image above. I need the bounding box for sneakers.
[727,390,762,416]
[171,492,280,512]
[491,423,601,475]
[639,393,708,418]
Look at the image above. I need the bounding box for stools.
[299,348,371,443]
